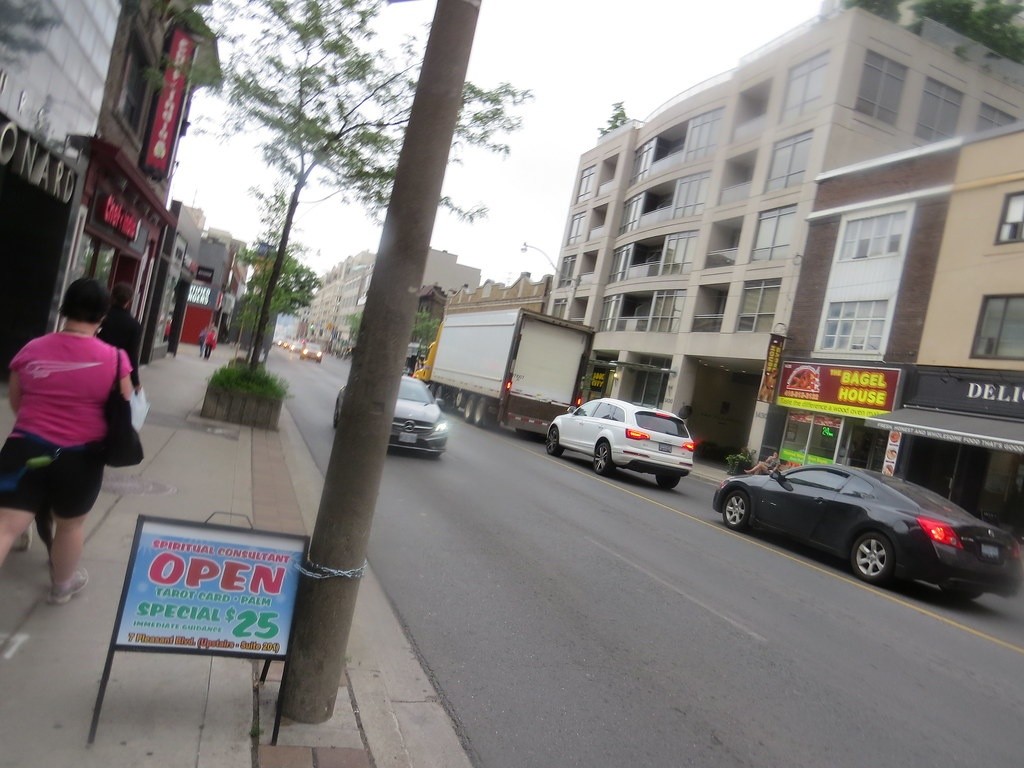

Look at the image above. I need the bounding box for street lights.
[519,246,583,320]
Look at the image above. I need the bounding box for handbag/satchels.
[100,348,144,466]
[129,385,150,432]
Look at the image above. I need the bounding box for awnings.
[864,405,1024,455]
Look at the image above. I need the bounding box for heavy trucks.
[410,307,598,444]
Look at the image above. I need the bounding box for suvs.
[544,398,697,492]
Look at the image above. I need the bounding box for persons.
[744,452,780,475]
[0,277,142,601]
[157,319,173,342]
[199,324,217,360]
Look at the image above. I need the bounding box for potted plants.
[725,452,753,476]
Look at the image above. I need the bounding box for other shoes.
[744,470,754,475]
[52,568,89,604]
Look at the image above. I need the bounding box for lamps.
[939,369,964,384]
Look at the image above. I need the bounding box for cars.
[275,335,304,354]
[333,371,448,461]
[299,342,322,364]
[713,463,1011,609]
